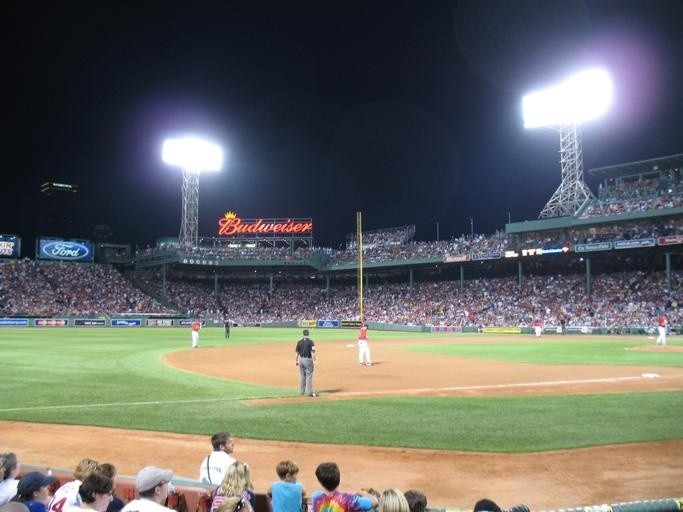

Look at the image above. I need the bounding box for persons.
[295,330,319,397]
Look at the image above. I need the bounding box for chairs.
[14,463,275,512]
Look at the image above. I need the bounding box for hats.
[135,467,174,492]
[17,471,57,497]
